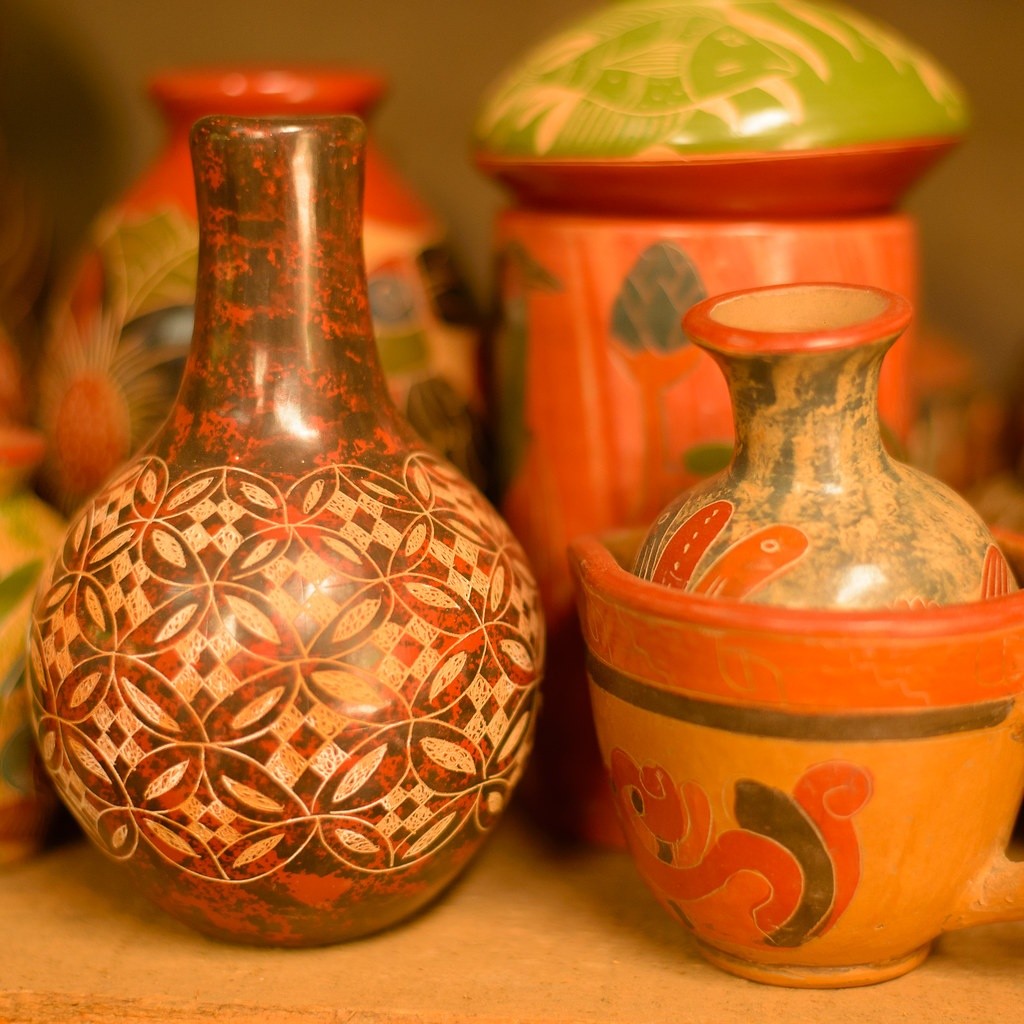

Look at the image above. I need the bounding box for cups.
[568,522,1023,992]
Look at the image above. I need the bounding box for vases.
[1,428,63,869]
[621,282,1020,610]
[33,67,487,512]
[26,117,549,945]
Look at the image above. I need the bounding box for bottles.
[26,113,547,950]
[633,279,1019,605]
